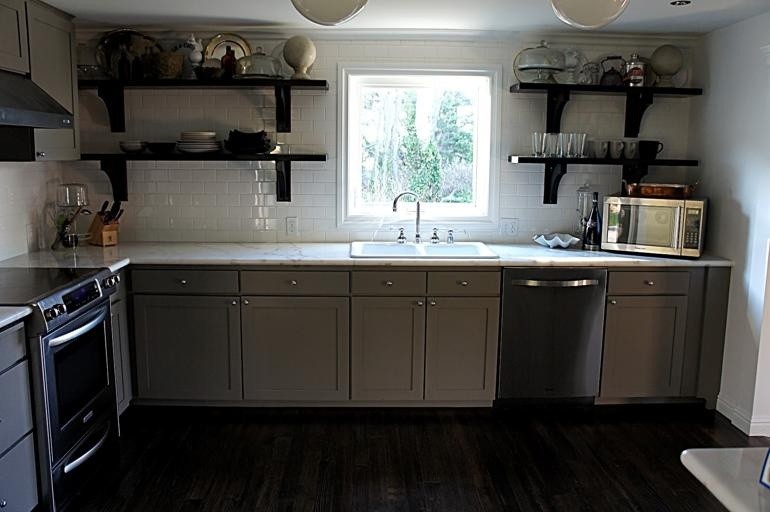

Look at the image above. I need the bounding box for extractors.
[0,69,76,130]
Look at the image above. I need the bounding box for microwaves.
[600,192,710,259]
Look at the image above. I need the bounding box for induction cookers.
[1,266,120,333]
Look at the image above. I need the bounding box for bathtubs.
[420,239,499,259]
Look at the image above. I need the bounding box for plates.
[514,48,588,85]
[120,129,276,154]
[95,29,152,81]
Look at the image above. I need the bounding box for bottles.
[574,183,593,247]
[220,45,236,80]
[625,52,648,87]
[582,191,602,251]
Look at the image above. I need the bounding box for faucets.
[391,190,422,242]
[447,229,455,244]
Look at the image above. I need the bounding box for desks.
[680,447,770,512]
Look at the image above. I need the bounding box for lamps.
[290,0,368,25]
[549,0,633,31]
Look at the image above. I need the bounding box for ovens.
[24,300,121,511]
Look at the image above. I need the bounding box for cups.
[530,132,588,158]
[587,138,663,161]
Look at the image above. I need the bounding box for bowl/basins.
[76,65,99,81]
[195,67,224,80]
[625,182,692,199]
[154,54,182,80]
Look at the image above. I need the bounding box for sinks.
[350,240,420,259]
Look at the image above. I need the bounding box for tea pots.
[184,34,204,65]
[598,54,630,88]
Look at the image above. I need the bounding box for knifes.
[101,199,123,224]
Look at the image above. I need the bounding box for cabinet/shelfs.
[0,0,31,75]
[0,322,39,512]
[26,0,81,161]
[594,267,731,409]
[109,266,135,418]
[348,264,503,408]
[126,264,351,407]
[75,77,329,202]
[508,82,704,204]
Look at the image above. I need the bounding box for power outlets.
[287,218,298,236]
[504,218,518,237]
